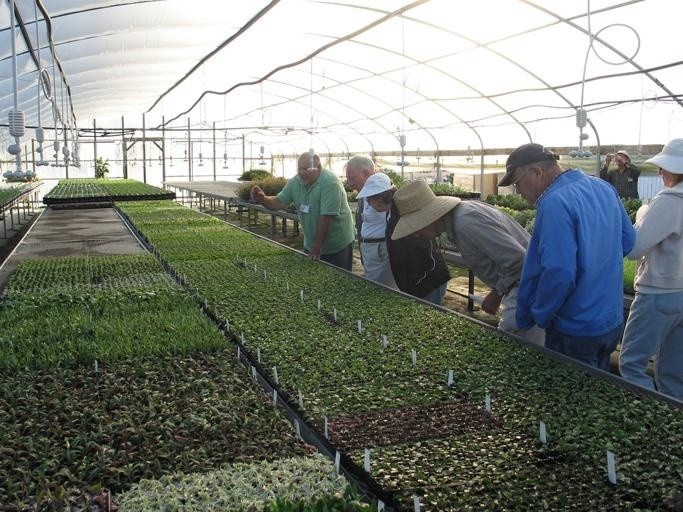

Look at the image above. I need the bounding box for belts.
[359,237,386,243]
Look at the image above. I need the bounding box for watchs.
[494,288,504,297]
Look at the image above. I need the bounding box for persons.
[353,172,451,306]
[599,149,642,199]
[344,155,399,291]
[251,152,354,272]
[389,178,545,349]
[615,138,682,401]
[496,143,636,372]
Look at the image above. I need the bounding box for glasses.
[512,167,532,188]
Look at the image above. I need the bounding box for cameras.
[607,154,615,159]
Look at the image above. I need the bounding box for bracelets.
[623,161,628,166]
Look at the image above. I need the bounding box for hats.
[644,136,683,175]
[390,176,462,242]
[616,150,631,163]
[355,173,395,200]
[495,142,561,186]
